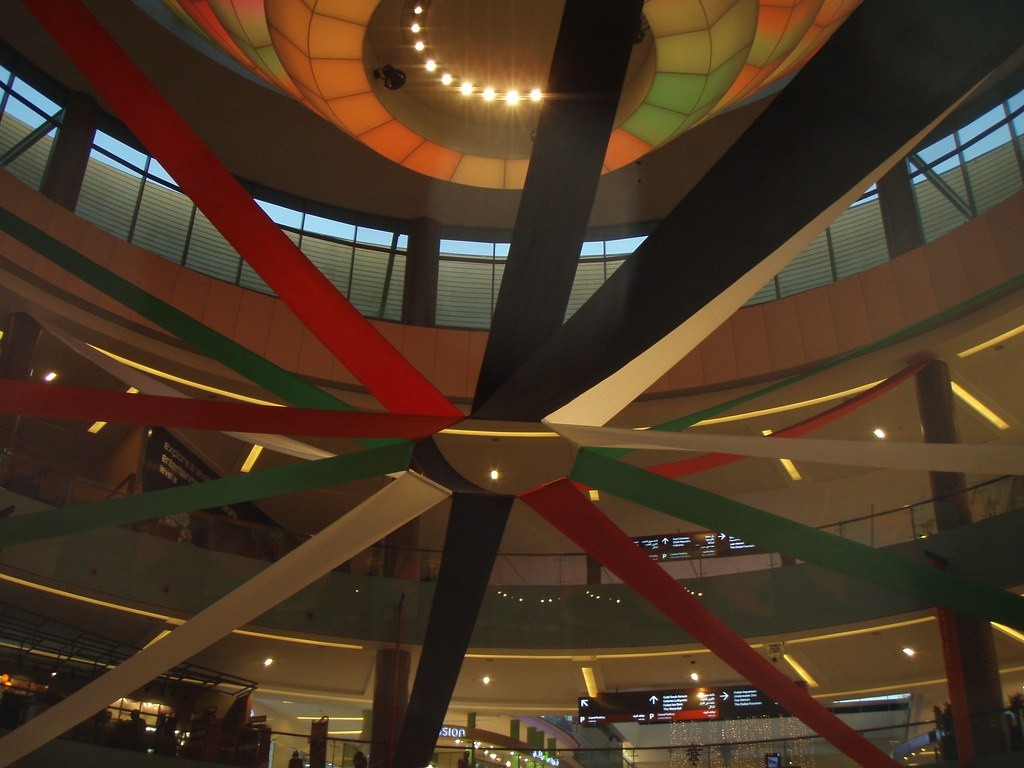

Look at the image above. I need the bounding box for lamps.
[374,64,407,91]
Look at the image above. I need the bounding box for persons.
[458,751,471,768]
[288,751,303,768]
[154,713,178,757]
[353,752,367,768]
[130,710,146,734]
